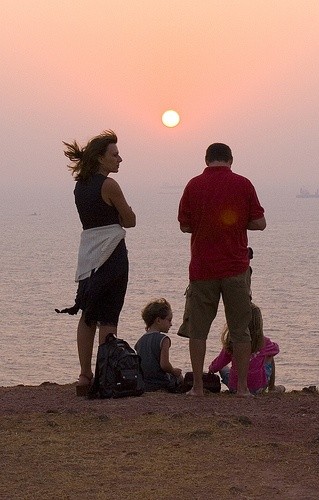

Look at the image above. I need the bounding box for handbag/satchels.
[183,371,222,393]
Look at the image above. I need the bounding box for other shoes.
[269,384,286,395]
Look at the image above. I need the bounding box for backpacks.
[88,333,146,399]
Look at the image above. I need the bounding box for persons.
[54,129,136,396]
[134,298,194,395]
[208,304,286,393]
[176,143,266,397]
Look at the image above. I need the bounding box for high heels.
[75,371,94,397]
